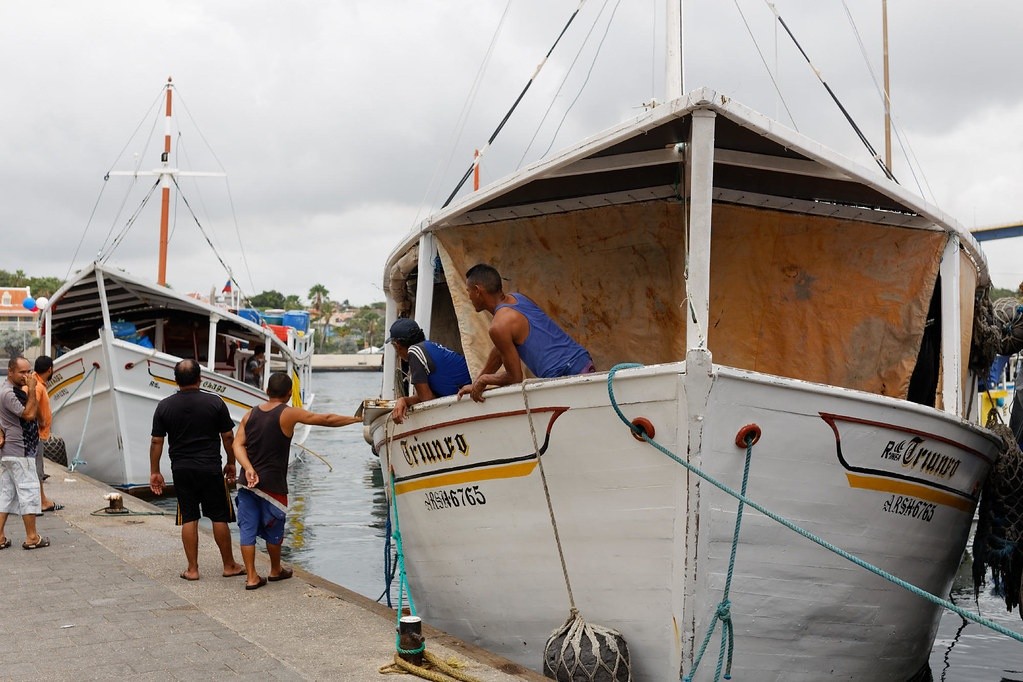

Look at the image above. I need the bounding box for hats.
[385,318,423,344]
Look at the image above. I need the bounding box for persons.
[385,319,472,426]
[150,359,247,583]
[245,345,267,389]
[232,372,362,590]
[0,356,65,549]
[457,263,596,403]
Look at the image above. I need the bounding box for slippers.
[180,570,200,580]
[244,575,267,589]
[22,535,50,550]
[268,566,293,581]
[223,565,247,577]
[43,502,64,512]
[0,536,12,549]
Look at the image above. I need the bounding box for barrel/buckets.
[101,321,137,343]
[239,309,260,324]
[128,336,154,349]
[263,309,310,335]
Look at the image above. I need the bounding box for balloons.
[23,297,48,312]
